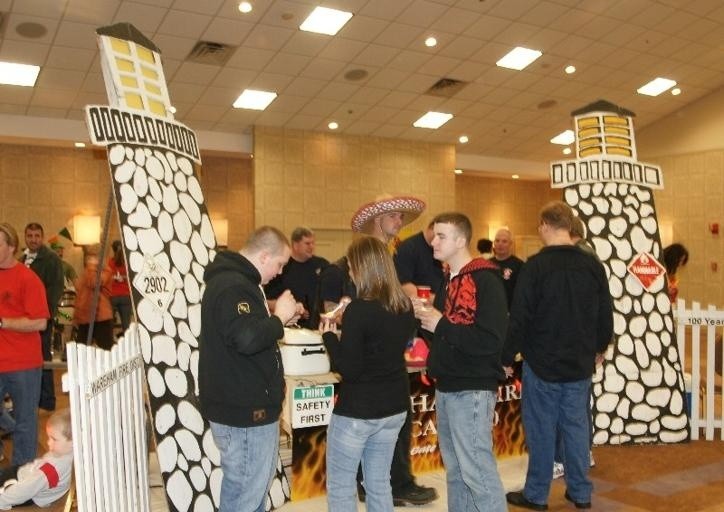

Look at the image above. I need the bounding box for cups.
[416,285,432,307]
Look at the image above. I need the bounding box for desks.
[278,357,524,506]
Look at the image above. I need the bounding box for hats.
[351,193,426,234]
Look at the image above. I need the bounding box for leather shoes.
[565,489,591,509]
[506,493,548,512]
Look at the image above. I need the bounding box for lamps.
[69,209,102,271]
[207,215,230,253]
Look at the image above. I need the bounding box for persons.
[393,218,444,292]
[0,222,48,467]
[502,199,613,511]
[17,223,65,416]
[345,210,437,507]
[0,407,74,510]
[409,212,515,512]
[315,236,416,511]
[661,242,688,308]
[197,226,305,511]
[105,240,132,331]
[265,228,331,330]
[476,228,524,287]
[73,254,114,351]
[552,214,603,481]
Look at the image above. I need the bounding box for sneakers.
[553,461,564,480]
[392,485,436,507]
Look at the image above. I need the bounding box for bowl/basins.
[320,313,339,324]
